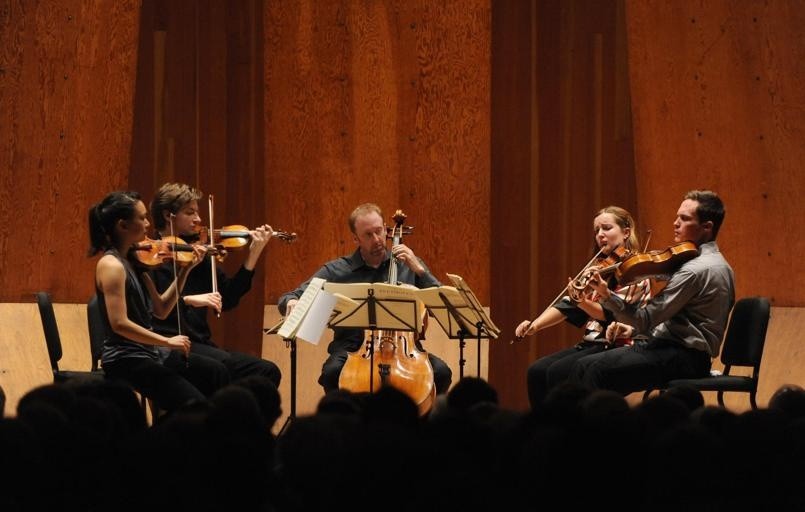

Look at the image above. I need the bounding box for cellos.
[338,210,437,416]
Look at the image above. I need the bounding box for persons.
[515,205,654,407]
[278,203,453,394]
[149,182,282,387]
[88,190,230,422]
[568,189,736,397]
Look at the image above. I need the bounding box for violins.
[127,234,228,269]
[566,241,698,305]
[179,224,297,252]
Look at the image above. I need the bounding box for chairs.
[35,289,146,425]
[642,293,771,412]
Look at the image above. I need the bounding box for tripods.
[276,338,297,439]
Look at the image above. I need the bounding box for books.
[324,282,423,334]
[277,277,327,341]
[415,285,499,339]
